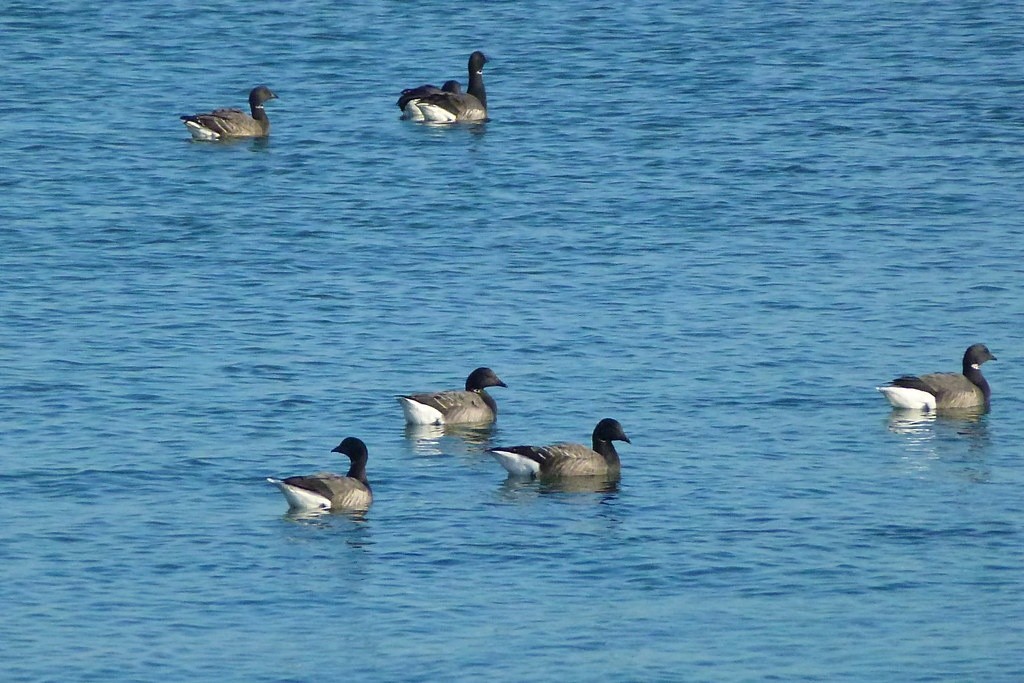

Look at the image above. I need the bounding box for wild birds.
[395,366,509,426]
[876,343,998,413]
[180,84,279,140]
[483,417,632,482]
[395,50,490,124]
[265,436,373,512]
